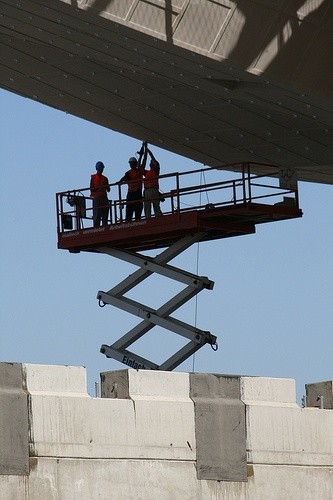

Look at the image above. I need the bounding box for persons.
[138,146,163,218]
[90,161,110,227]
[118,141,148,222]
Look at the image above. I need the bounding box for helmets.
[96,161,104,169]
[128,157,137,163]
